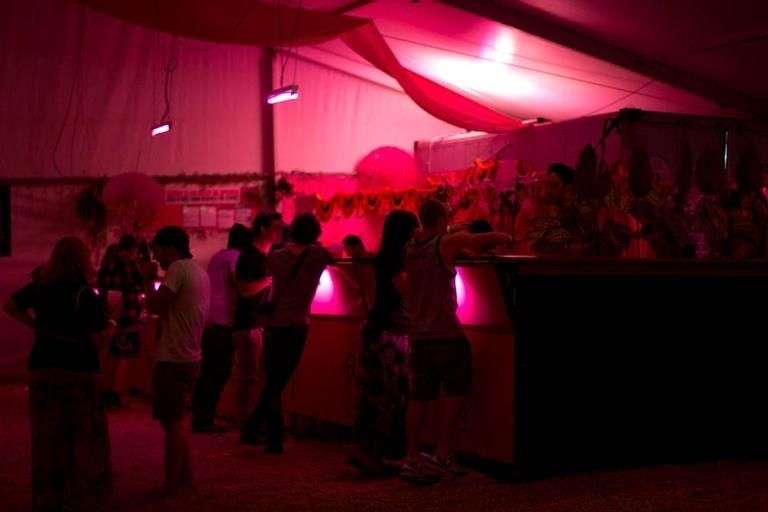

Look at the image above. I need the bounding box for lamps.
[265,85,298,104]
[149,121,173,135]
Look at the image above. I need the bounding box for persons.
[450,186,482,227]
[1,236,124,511]
[513,136,767,264]
[98,207,342,497]
[489,189,520,231]
[343,199,514,476]
